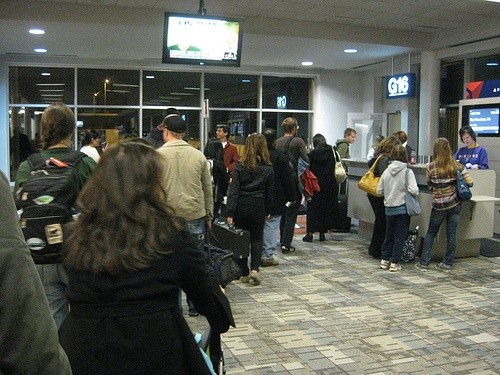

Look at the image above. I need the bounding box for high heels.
[281,245,295,254]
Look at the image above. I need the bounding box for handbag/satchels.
[399,228,419,263]
[359,154,387,197]
[405,168,422,216]
[203,229,241,290]
[194,327,226,375]
[332,147,346,183]
[454,169,472,201]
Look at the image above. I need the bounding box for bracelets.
[465,141,476,162]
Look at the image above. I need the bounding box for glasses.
[294,124,300,129]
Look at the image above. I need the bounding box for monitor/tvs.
[462,103,500,137]
[162,12,244,68]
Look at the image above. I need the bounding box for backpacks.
[15,149,87,265]
[277,136,296,179]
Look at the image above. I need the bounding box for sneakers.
[389,263,403,272]
[415,262,427,271]
[435,263,452,272]
[379,260,390,269]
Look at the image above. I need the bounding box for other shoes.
[303,233,313,242]
[250,270,261,284]
[294,224,302,228]
[369,251,382,258]
[241,270,251,283]
[319,234,325,241]
[261,257,279,266]
[188,308,200,316]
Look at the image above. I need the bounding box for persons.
[0,171,72,375]
[9,103,490,331]
[62,138,236,375]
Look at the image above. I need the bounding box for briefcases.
[205,218,250,259]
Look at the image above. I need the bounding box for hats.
[157,115,187,133]
